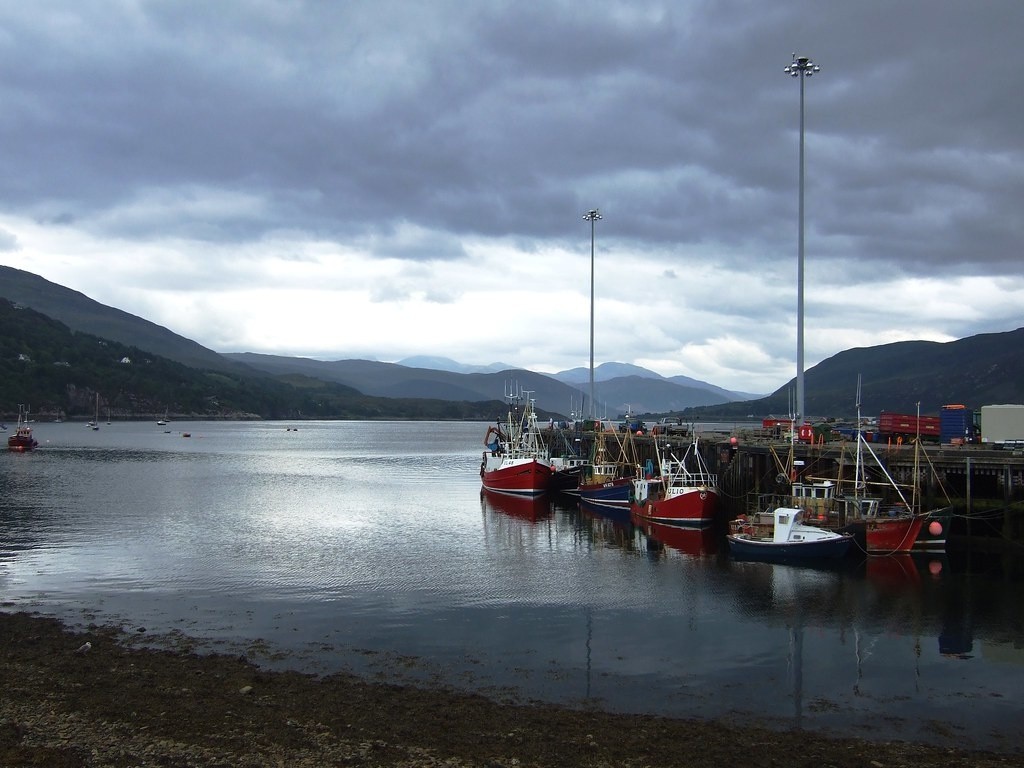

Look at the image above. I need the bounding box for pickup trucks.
[618,418,647,435]
[651,417,688,437]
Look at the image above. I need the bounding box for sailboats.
[163,407,170,422]
[85,392,112,430]
[157,408,166,425]
[54,412,61,423]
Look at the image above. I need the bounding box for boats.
[749,370,932,556]
[183,432,190,437]
[581,417,634,512]
[631,436,723,532]
[0,424,7,433]
[164,431,171,433]
[861,402,955,553]
[480,378,554,496]
[24,420,35,422]
[549,436,589,499]
[725,507,856,563]
[481,485,556,526]
[7,403,38,451]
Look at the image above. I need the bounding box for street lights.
[784,54,822,426]
[583,210,603,417]
